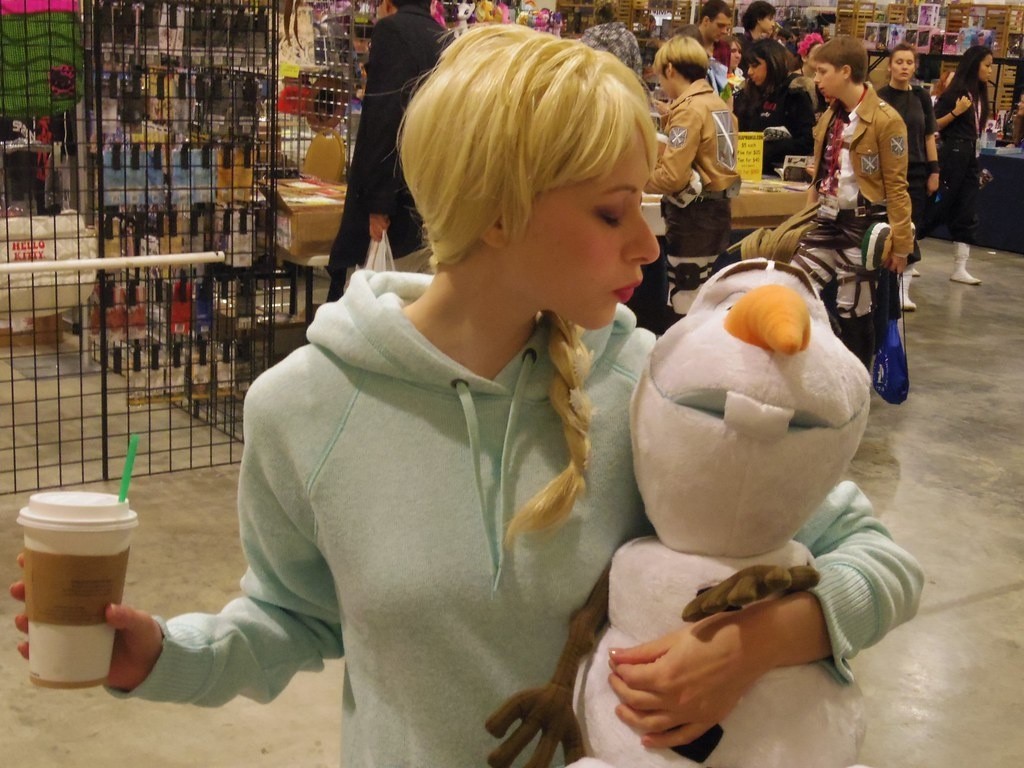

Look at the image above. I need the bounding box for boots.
[950,240,982,284]
[897,265,917,311]
[913,268,920,276]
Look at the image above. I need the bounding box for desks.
[925,145,1024,256]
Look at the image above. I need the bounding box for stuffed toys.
[430,0,563,37]
[486,259,868,768]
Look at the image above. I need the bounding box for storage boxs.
[641,193,667,236]
[254,178,346,258]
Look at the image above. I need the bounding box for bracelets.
[951,111,957,118]
[927,161,940,173]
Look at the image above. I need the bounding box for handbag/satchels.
[365,227,396,274]
[872,319,910,404]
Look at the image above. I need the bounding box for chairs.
[301,129,344,182]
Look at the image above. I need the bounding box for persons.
[326,0,455,302]
[575,0,994,379]
[10,24,923,768]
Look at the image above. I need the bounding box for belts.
[700,190,726,201]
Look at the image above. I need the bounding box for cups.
[17,491,139,690]
